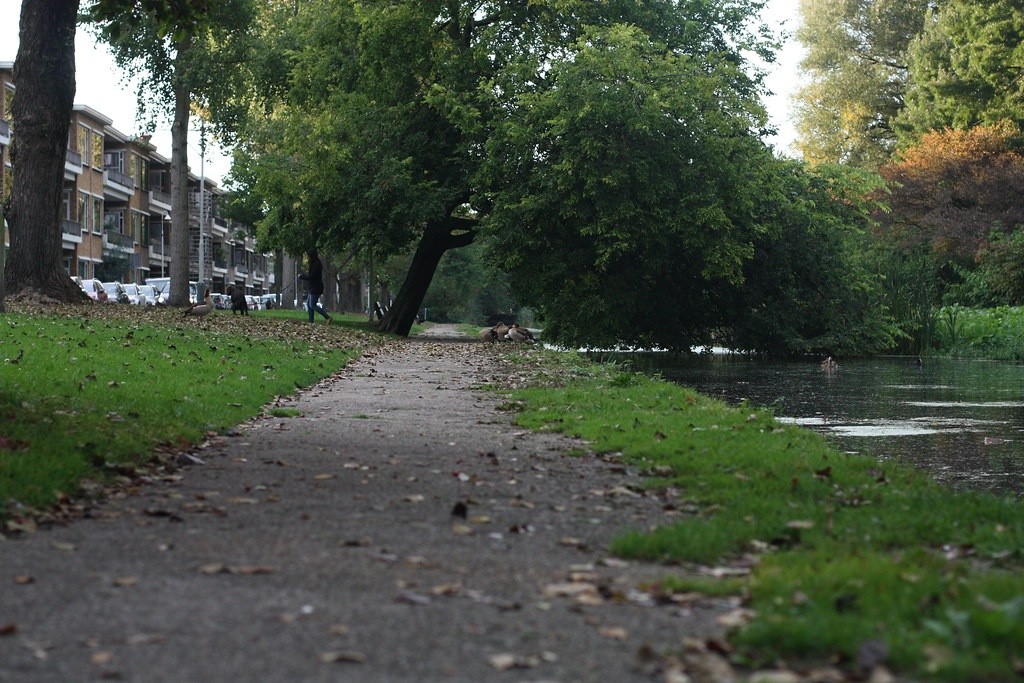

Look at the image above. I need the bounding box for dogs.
[225,285,249,316]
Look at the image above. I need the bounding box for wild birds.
[180,287,216,322]
[478,321,540,345]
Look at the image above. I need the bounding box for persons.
[298,247,333,325]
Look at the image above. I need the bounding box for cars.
[69,275,322,311]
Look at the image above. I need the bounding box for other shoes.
[326,317,333,325]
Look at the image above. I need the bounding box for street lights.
[161,211,172,278]
